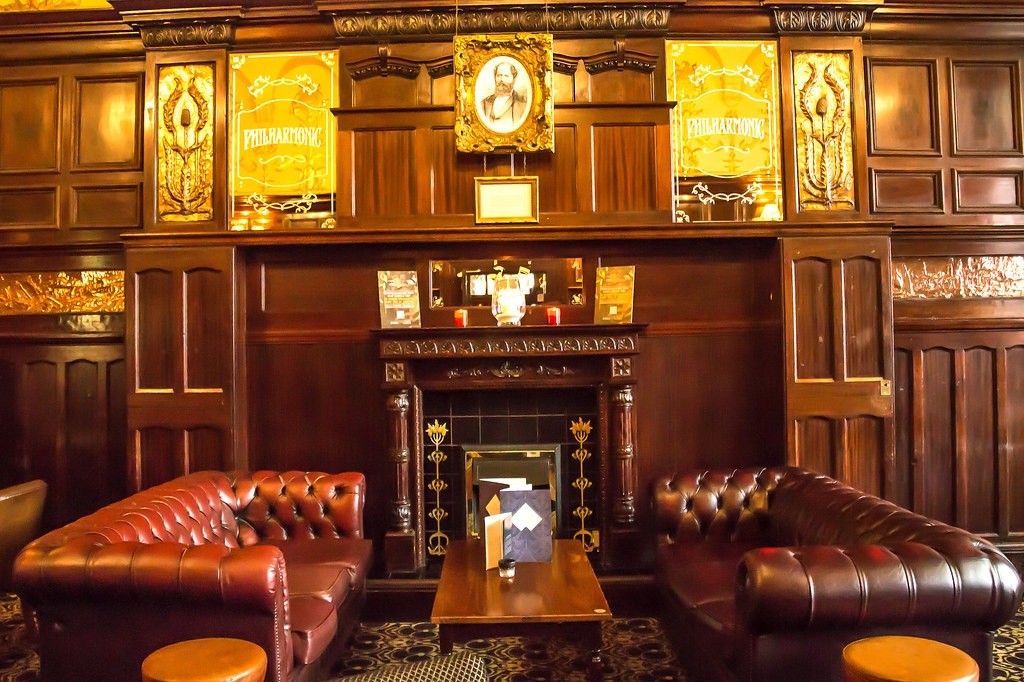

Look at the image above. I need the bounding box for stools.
[138,635,266,682]
[841,634,979,682]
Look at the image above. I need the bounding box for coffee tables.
[430,538,615,682]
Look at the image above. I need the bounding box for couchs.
[9,468,373,682]
[644,466,1024,682]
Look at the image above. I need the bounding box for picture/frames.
[452,31,555,155]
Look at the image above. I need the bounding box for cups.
[498,559,516,577]
